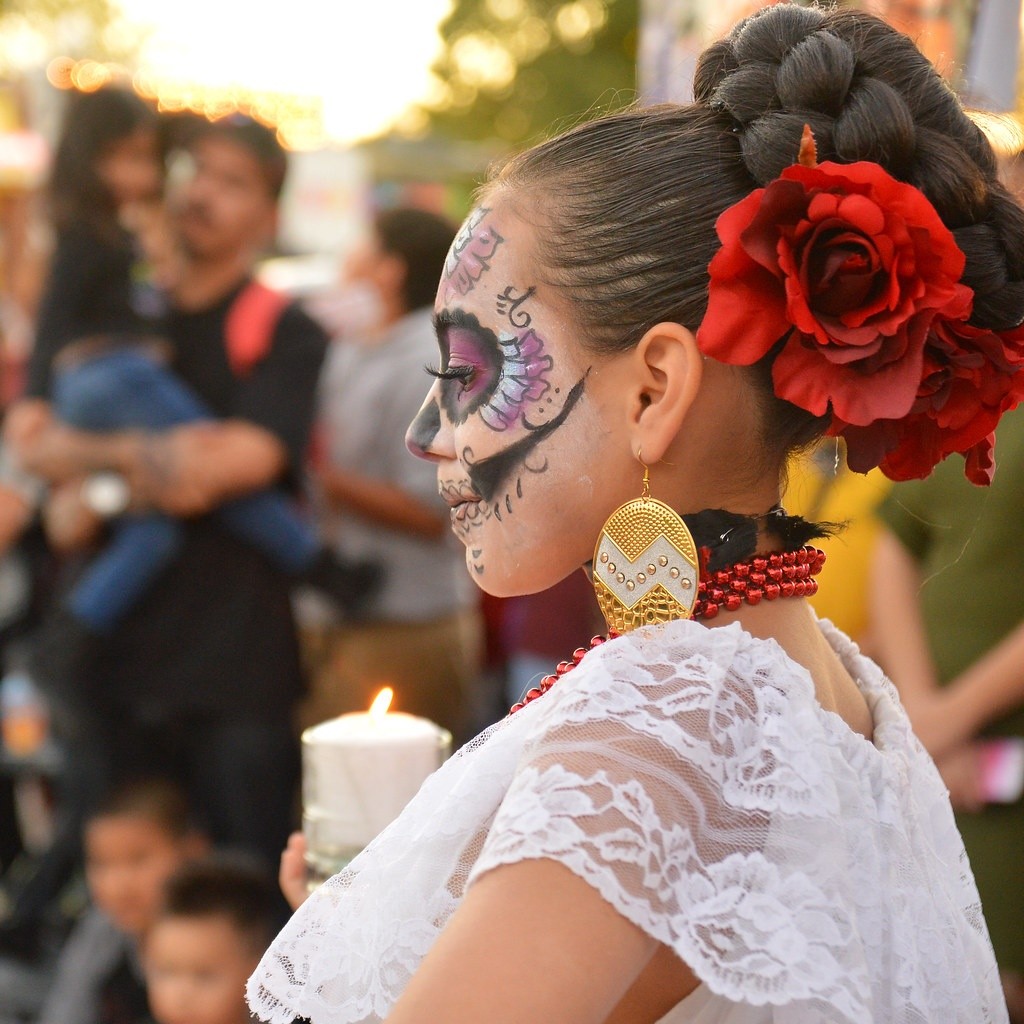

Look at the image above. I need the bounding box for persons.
[301,207,484,737]
[478,567,595,711]
[780,433,1024,805]
[246,6,1023,1023]
[0,82,324,1024]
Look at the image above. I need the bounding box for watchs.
[85,469,129,540]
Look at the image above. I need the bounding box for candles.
[300,686,452,896]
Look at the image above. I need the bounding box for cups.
[300,717,454,894]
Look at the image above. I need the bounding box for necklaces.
[508,503,826,714]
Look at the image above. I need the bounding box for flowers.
[696,124,974,426]
[822,325,1024,488]
